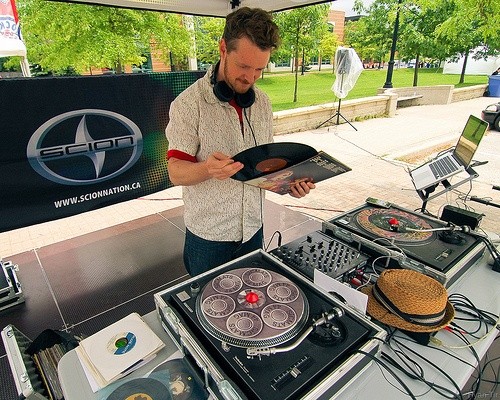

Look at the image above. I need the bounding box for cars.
[408,59,423,68]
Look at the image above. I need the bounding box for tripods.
[316,75,359,132]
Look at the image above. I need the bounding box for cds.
[75,312,166,393]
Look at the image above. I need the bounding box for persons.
[492,67,500,75]
[164,7,315,278]
[418,61,430,68]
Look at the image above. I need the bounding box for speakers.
[337,49,351,74]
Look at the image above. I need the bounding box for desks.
[57,247,500,400]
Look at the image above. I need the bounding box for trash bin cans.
[426,63,429,68]
[488,74,500,97]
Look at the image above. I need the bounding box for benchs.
[397,95,423,102]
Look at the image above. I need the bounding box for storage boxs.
[0,261,25,311]
[1,324,50,400]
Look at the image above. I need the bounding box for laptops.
[410,114,490,193]
